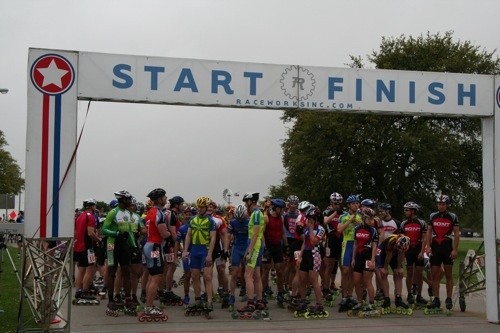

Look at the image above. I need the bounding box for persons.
[360,199,384,301]
[375,233,410,308]
[399,201,428,305]
[323,192,344,301]
[351,207,379,311]
[426,195,460,310]
[1,188,327,316]
[337,195,365,306]
[374,203,401,301]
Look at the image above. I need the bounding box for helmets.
[330,192,343,203]
[361,207,375,219]
[377,203,391,209]
[83,198,98,205]
[114,190,132,199]
[196,196,210,207]
[403,202,420,208]
[297,201,310,209]
[346,195,360,203]
[305,208,321,219]
[361,199,374,205]
[167,196,184,204]
[146,188,166,199]
[234,205,247,220]
[270,198,286,207]
[287,195,299,203]
[434,195,449,203]
[242,192,259,201]
[396,236,410,253]
[111,199,234,217]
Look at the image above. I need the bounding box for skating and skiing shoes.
[70,274,454,323]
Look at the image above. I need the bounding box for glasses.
[334,203,342,206]
